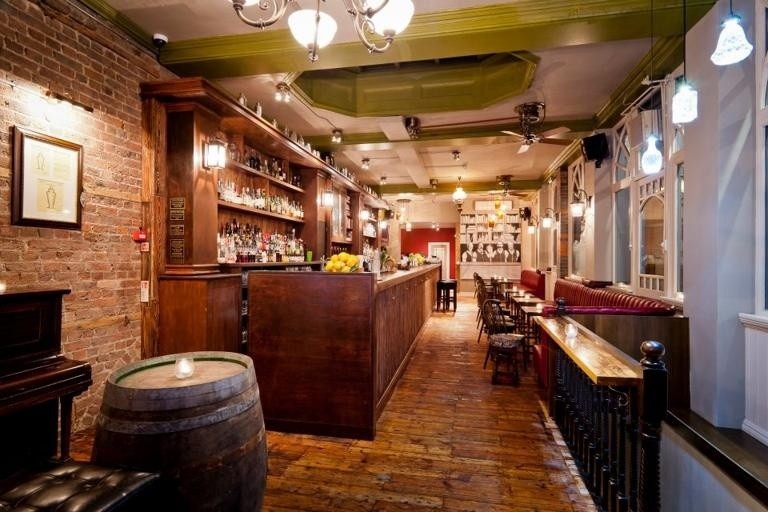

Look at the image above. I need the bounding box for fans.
[488,103,575,156]
[403,116,434,141]
[486,175,529,198]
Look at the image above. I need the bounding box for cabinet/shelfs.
[332,182,394,257]
[220,156,307,272]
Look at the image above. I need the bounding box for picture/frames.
[9,124,85,231]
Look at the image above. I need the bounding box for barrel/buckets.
[89,350,269,511]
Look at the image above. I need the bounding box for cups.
[306,250,313,262]
[565,323,578,336]
[174,354,195,379]
[566,338,578,349]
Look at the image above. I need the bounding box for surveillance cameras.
[153,34,168,47]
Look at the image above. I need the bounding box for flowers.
[324,251,362,274]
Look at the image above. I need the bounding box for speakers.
[519,207,528,217]
[580,133,609,162]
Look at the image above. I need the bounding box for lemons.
[325,252,358,273]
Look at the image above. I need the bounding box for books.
[460,214,520,243]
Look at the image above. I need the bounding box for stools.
[1,459,162,511]
[436,278,458,314]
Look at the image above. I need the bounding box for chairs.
[470,269,676,385]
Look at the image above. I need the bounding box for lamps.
[272,81,390,186]
[451,176,467,200]
[203,137,230,173]
[228,0,416,61]
[636,1,751,176]
[320,187,335,208]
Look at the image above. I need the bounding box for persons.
[462,242,520,262]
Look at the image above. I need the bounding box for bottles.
[237,92,246,106]
[372,251,382,281]
[255,101,263,117]
[285,127,322,159]
[217,141,304,263]
[273,119,278,127]
